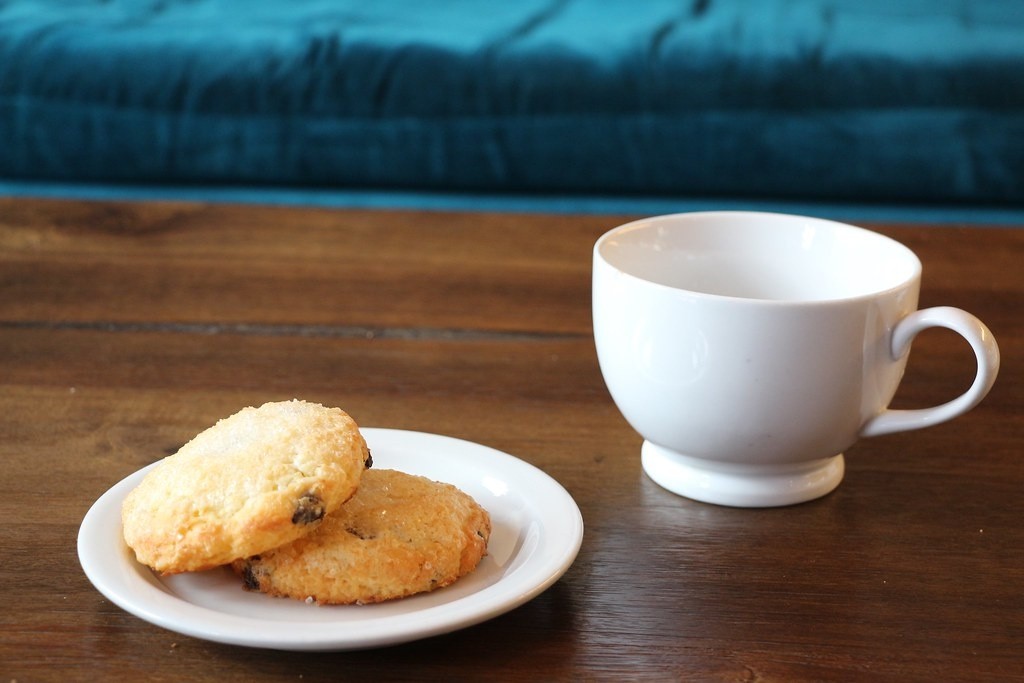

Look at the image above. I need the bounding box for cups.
[592,208,1000,507]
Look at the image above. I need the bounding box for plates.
[77,425,584,652]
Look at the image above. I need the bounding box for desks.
[0,197,1024,683]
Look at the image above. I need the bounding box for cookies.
[121,398,373,575]
[230,466,491,604]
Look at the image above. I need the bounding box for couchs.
[0,0,1024,220]
